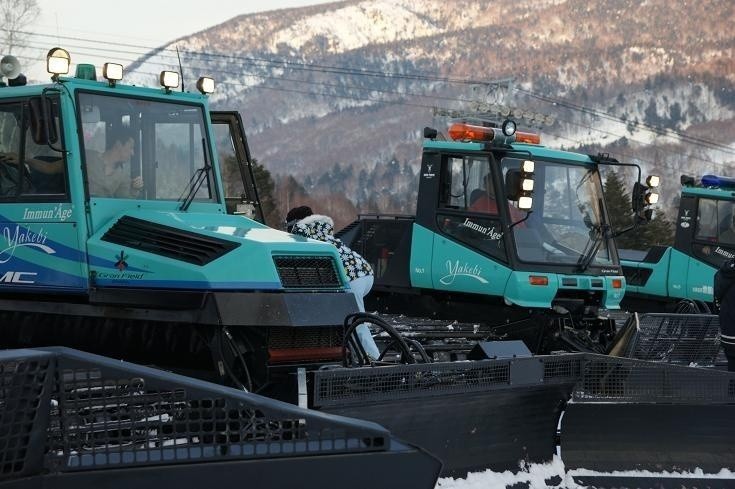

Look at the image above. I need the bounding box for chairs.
[30,156,63,191]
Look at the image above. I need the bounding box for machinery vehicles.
[598,173,735,319]
[331,118,626,337]
[2,46,366,381]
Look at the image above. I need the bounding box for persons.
[283,205,387,363]
[0,123,144,197]
[465,169,529,228]
